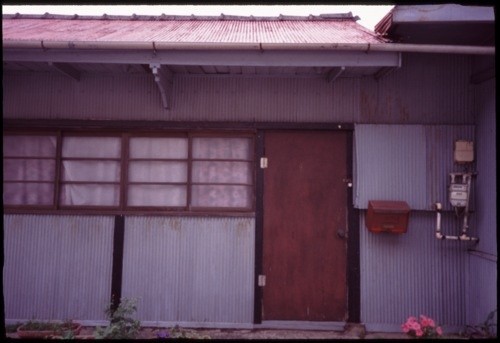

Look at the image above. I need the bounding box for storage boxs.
[365,200,411,233]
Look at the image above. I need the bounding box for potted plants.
[17,321,82,340]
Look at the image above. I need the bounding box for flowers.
[402,313,443,338]
